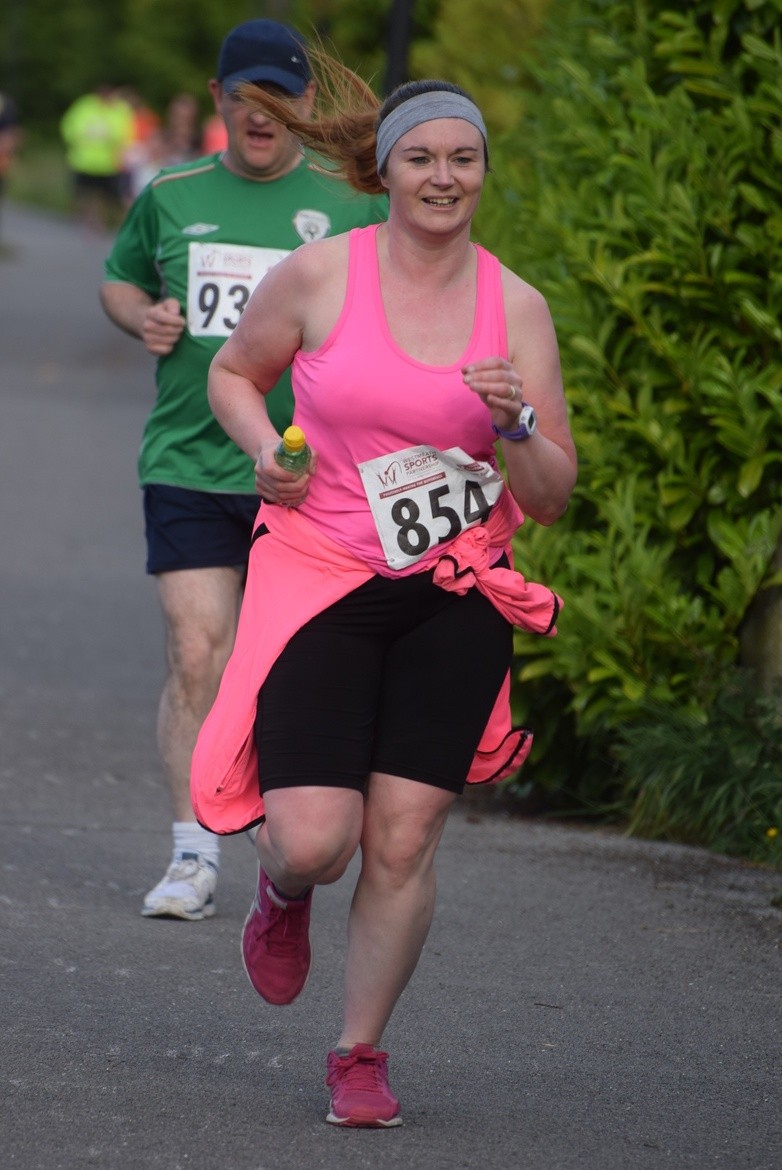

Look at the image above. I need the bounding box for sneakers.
[325,1042,403,1128]
[239,858,314,1005]
[142,849,214,921]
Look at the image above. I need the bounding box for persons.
[61,82,229,237]
[100,21,389,921]
[0,92,20,172]
[191,82,579,1127]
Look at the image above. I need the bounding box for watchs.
[492,402,536,440]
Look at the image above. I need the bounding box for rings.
[506,385,516,400]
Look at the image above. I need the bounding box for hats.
[219,18,311,97]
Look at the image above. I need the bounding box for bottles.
[273,425,312,508]
